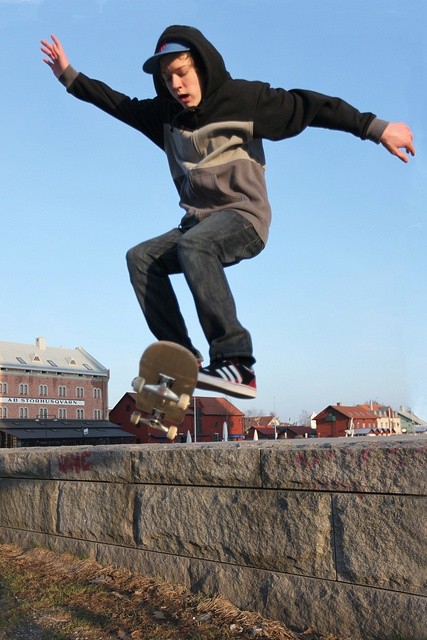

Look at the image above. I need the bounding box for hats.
[141,39,196,74]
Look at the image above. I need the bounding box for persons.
[40,25,415,399]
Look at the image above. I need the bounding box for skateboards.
[130,341,198,440]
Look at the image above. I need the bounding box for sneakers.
[196,357,258,399]
